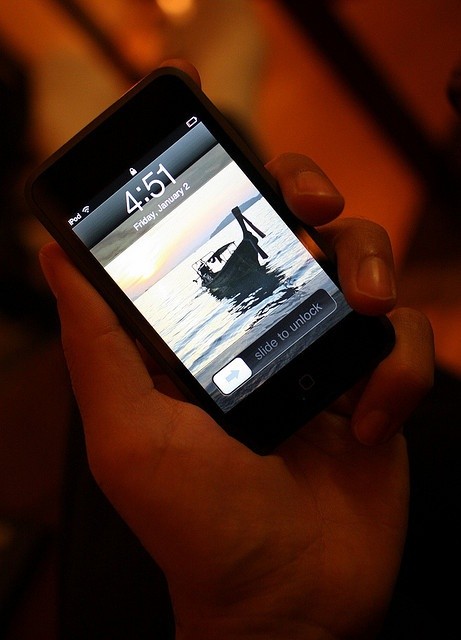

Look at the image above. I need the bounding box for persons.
[39,56,435,639]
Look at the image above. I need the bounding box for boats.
[191,206,268,288]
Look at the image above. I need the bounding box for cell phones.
[24,66,397,456]
